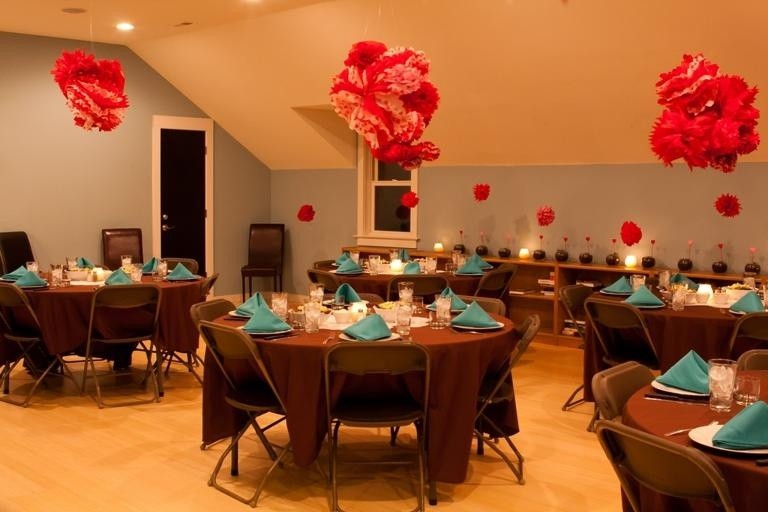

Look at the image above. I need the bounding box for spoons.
[664,420,719,437]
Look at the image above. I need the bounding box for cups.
[287,309,305,331]
[633,275,646,292]
[304,282,325,333]
[685,288,729,305]
[734,374,761,407]
[348,248,467,280]
[332,311,349,324]
[271,292,288,321]
[671,284,686,312]
[395,281,425,336]
[708,358,738,413]
[26,256,168,289]
[743,278,755,288]
[428,294,452,330]
[762,284,768,308]
[659,272,669,293]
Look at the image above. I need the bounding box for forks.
[322,333,335,344]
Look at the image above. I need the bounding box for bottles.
[357,309,364,321]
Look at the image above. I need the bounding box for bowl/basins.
[373,302,416,323]
[725,286,759,303]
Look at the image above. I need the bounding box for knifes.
[645,392,709,404]
[223,318,250,320]
[264,333,299,340]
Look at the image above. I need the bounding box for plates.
[163,274,201,281]
[228,310,251,318]
[452,322,504,330]
[687,424,768,454]
[729,310,768,315]
[426,302,470,313]
[455,271,484,276]
[322,298,369,306]
[331,262,340,267]
[237,325,294,335]
[600,289,630,295]
[338,332,400,343]
[635,304,665,309]
[482,266,494,270]
[650,379,709,398]
[0,278,17,282]
[15,284,49,289]
[329,270,362,275]
